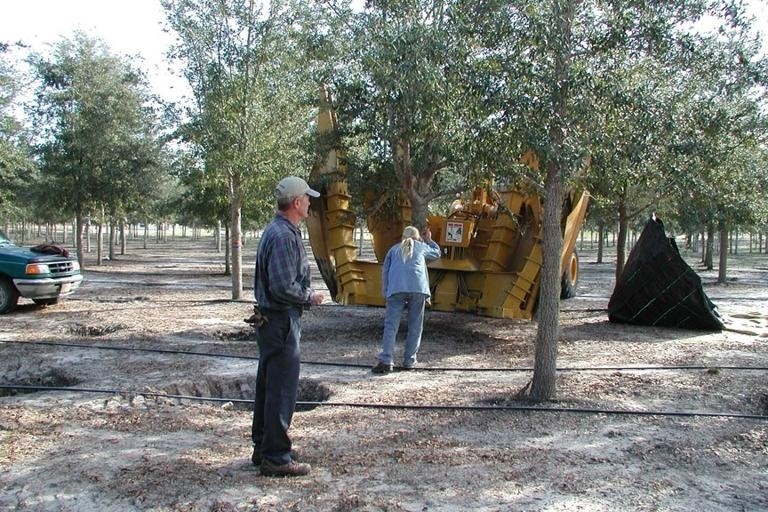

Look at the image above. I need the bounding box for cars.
[0,228,84,315]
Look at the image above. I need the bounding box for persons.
[251,176,324,475]
[372,226,441,373]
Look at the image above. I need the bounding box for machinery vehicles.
[303,83,590,320]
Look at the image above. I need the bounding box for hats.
[274,177,320,199]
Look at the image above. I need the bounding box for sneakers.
[370,362,392,373]
[252,444,311,476]
[404,360,419,370]
